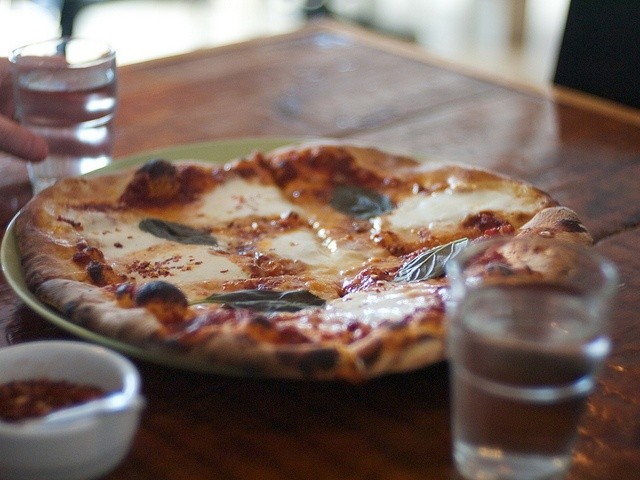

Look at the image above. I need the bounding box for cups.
[7,37,116,199]
[445,234,618,480]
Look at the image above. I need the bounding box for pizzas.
[14,139,594,380]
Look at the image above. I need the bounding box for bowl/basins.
[0,340,143,480]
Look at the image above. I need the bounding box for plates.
[1,134,453,379]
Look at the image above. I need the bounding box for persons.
[1,53,69,164]
